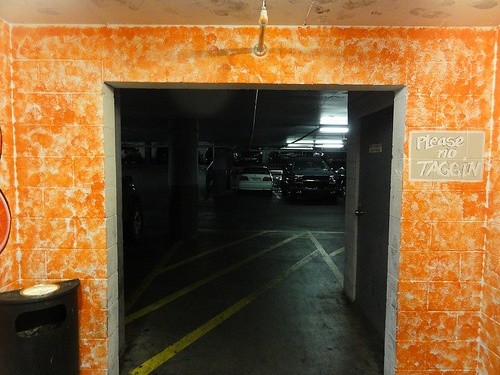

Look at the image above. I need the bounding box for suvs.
[282,156,337,202]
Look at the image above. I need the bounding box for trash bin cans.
[1,278,81,374]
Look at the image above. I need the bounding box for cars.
[122,175,145,244]
[238,165,274,196]
